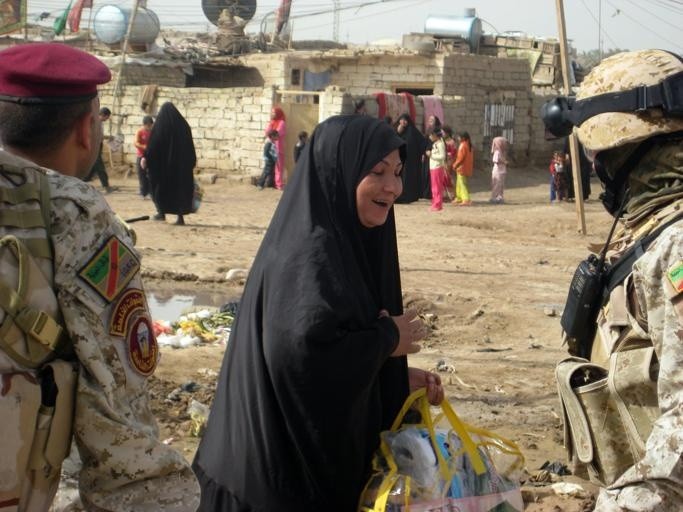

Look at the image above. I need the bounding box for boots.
[170,215,185,226]
[148,210,166,222]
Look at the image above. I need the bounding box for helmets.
[571,47,683,154]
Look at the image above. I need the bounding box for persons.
[139,102,196,226]
[353,102,594,212]
[191,113,445,511]
[540,48,683,512]
[264,107,286,190]
[84,107,118,195]
[1,43,112,184]
[1,145,202,512]
[292,131,307,164]
[133,115,154,200]
[256,129,280,191]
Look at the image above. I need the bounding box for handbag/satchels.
[356,422,530,512]
[191,181,205,214]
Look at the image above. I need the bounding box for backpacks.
[0,161,79,512]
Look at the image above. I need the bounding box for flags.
[52,0,74,36]
[68,0,93,32]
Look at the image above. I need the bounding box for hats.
[0,41,113,106]
